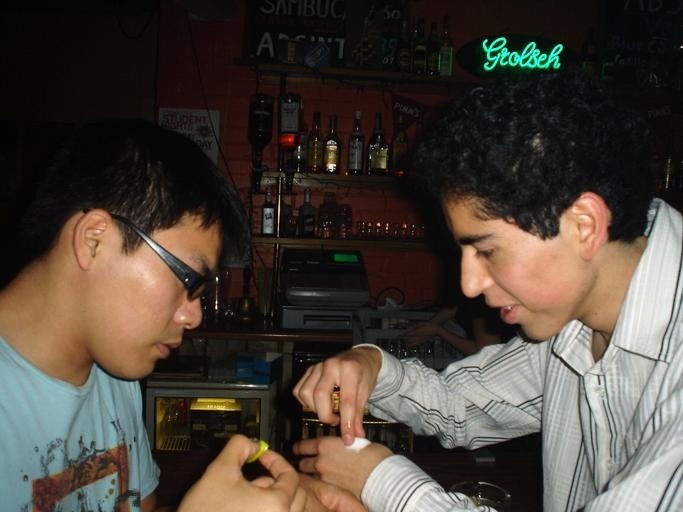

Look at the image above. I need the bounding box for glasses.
[81,206,210,301]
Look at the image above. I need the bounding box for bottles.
[246,91,409,177]
[411,12,455,78]
[260,182,315,237]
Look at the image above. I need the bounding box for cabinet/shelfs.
[181,55,485,345]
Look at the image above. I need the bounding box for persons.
[0,116,367,512]
[291,76,683,512]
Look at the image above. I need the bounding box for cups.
[314,191,354,239]
[449,478,514,511]
